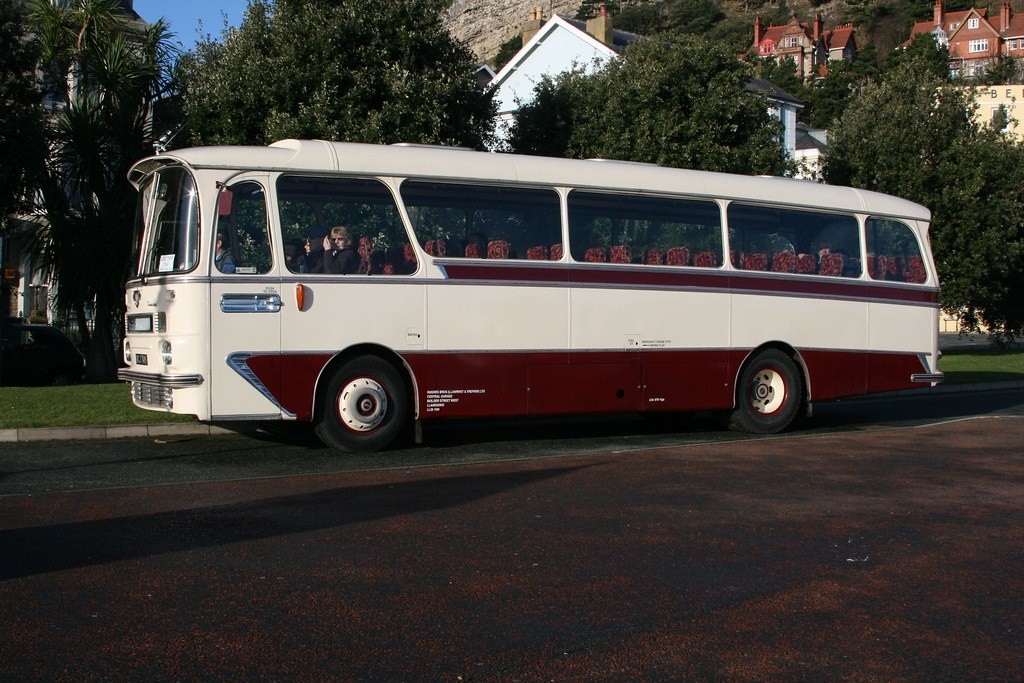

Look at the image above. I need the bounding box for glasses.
[330,237,346,242]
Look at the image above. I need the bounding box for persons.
[283,226,366,274]
[215,233,236,274]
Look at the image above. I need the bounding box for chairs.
[356,237,928,285]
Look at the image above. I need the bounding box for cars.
[0,321,87,386]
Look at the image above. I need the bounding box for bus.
[113,136,945,453]
